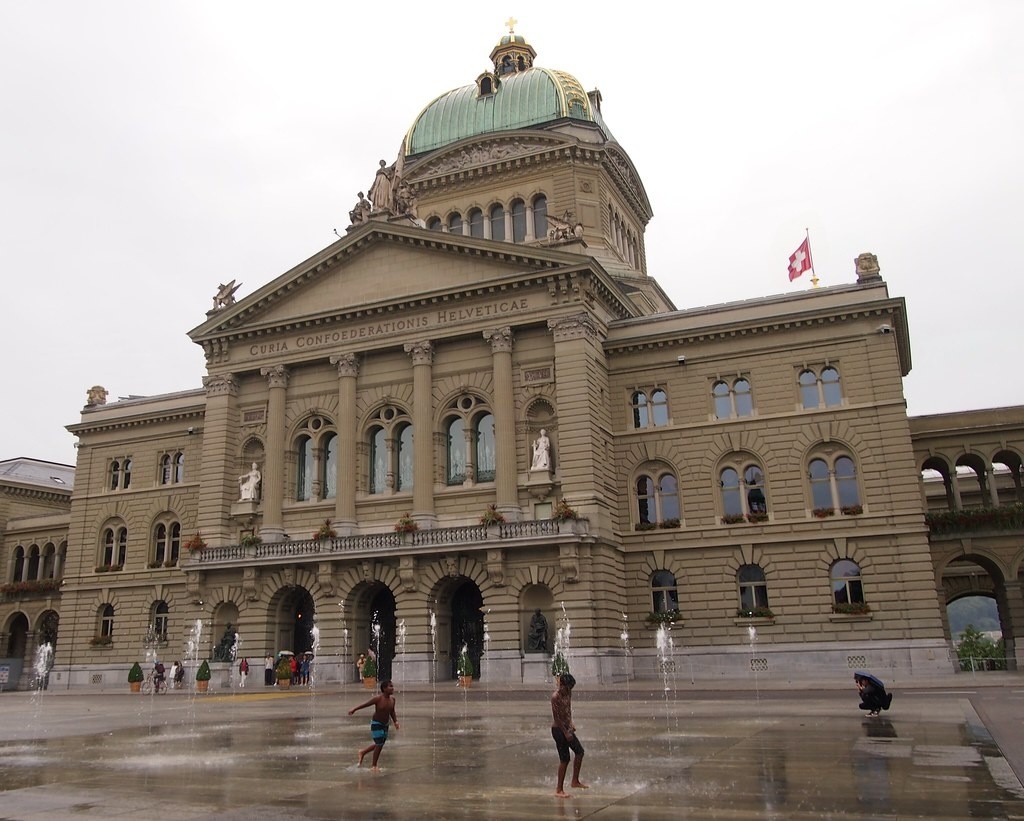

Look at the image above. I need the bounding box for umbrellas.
[854,672,885,691]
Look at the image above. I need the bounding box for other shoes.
[865,710,879,717]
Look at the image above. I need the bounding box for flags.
[788,238,810,282]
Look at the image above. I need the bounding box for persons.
[348,678,400,769]
[551,674,589,798]
[147,660,164,693]
[238,657,248,687]
[357,653,367,683]
[367,160,396,209]
[217,623,236,660]
[394,179,416,214]
[265,649,312,686]
[175,660,184,689]
[239,462,261,500]
[852,670,893,718]
[531,428,551,468]
[170,661,178,689]
[350,191,371,223]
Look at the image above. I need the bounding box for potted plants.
[552,652,569,686]
[362,655,376,689]
[660,517,681,529]
[92,635,112,645]
[277,657,290,690]
[128,662,145,692]
[196,660,211,692]
[164,560,175,567]
[149,559,160,568]
[243,535,262,548]
[458,653,472,689]
[635,522,656,530]
[95,565,108,573]
[647,610,680,623]
[144,632,166,643]
[111,564,121,572]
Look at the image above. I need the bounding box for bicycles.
[142,671,168,695]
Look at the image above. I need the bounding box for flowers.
[315,518,338,539]
[844,504,863,516]
[720,513,745,524]
[738,606,774,618]
[746,510,768,523]
[0,578,63,594]
[185,531,208,553]
[831,601,869,615]
[552,499,577,520]
[481,505,505,526]
[395,512,419,533]
[812,506,835,519]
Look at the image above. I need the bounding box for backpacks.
[873,685,892,710]
